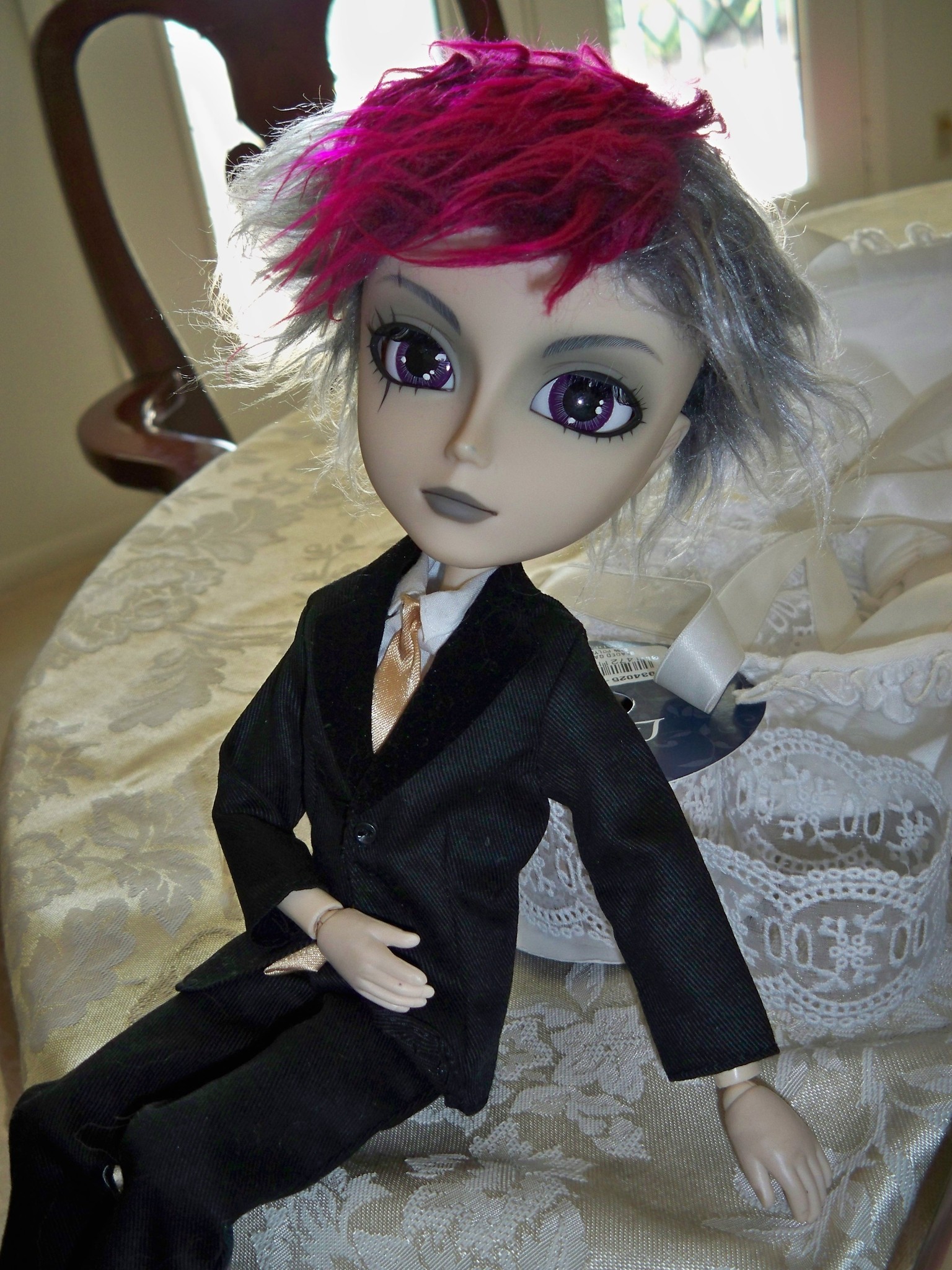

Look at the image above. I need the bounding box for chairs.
[34,0,516,488]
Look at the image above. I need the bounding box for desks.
[1,168,951,1270]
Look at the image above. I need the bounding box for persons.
[2,39,834,1270]
